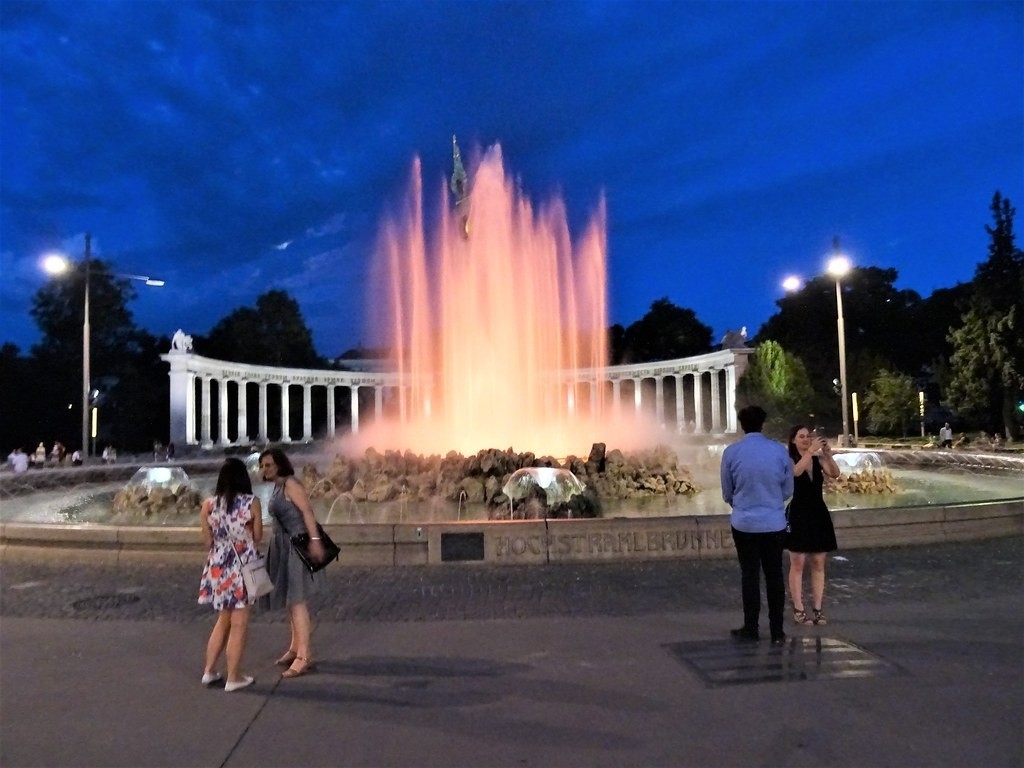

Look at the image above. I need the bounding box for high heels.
[282,657,317,677]
[278,650,297,665]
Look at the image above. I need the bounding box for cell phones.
[816,424,826,446]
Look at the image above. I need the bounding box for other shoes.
[224,676,254,692]
[202,672,222,685]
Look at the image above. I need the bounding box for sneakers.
[771,632,785,643]
[731,628,760,642]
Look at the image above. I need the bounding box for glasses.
[259,463,276,469]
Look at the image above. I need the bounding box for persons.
[956,432,970,447]
[198,457,274,691]
[974,431,1002,449]
[940,422,952,448]
[785,425,840,626]
[259,448,324,678]
[154,440,175,462]
[721,404,794,644]
[8,441,116,472]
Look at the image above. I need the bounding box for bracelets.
[309,537,321,540]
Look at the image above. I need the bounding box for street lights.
[40,232,167,459]
[779,234,851,447]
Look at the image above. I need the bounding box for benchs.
[857,442,1024,456]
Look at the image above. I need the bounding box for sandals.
[794,608,813,625]
[813,609,827,626]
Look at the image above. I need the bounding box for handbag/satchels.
[243,558,275,601]
[291,523,341,582]
